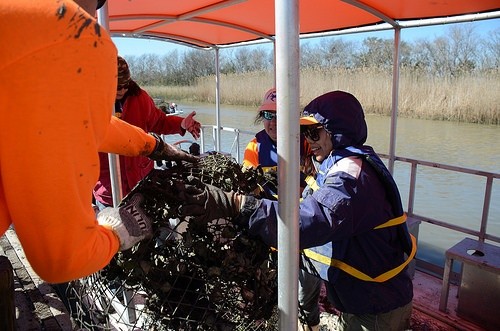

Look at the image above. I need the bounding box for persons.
[0,0,318,284]
[180,90,417,331]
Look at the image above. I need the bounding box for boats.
[154,98,184,117]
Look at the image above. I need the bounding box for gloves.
[95,193,154,252]
[147,132,200,168]
[176,175,241,226]
[181,110,203,140]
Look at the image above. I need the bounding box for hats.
[118,57,131,85]
[259,88,276,112]
[300,114,320,125]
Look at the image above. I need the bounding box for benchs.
[439,237,500,313]
[405,216,422,281]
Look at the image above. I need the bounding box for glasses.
[303,126,323,141]
[263,111,276,120]
[117,84,128,91]
